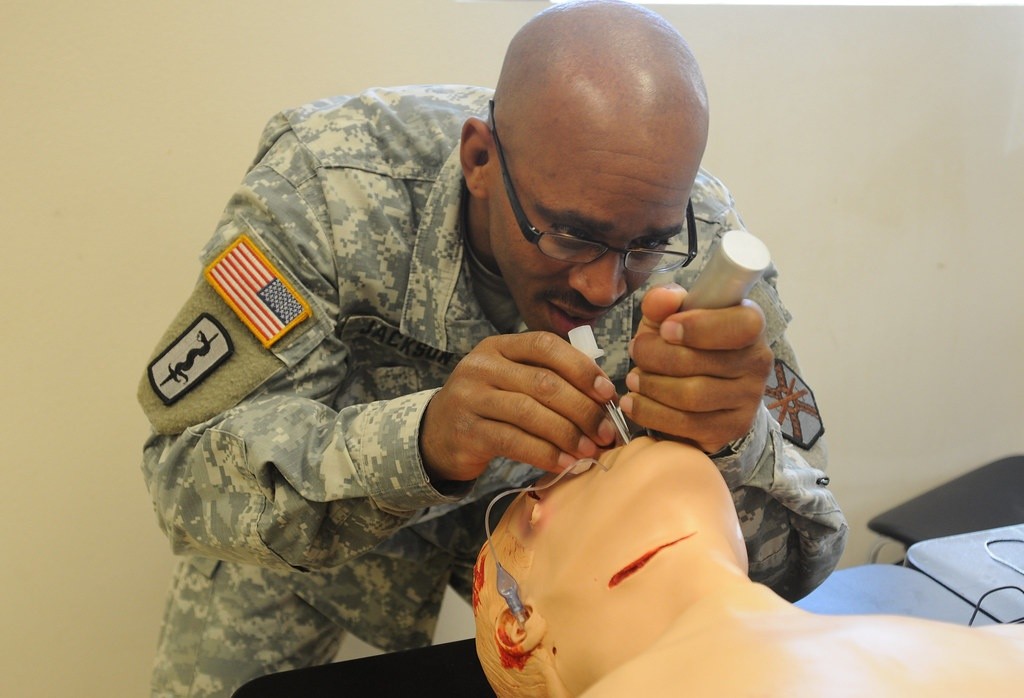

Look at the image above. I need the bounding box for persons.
[139,0,849,698]
[474,436,1024,698]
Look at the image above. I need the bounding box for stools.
[869,451,1024,567]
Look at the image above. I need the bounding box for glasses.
[487,100,697,274]
